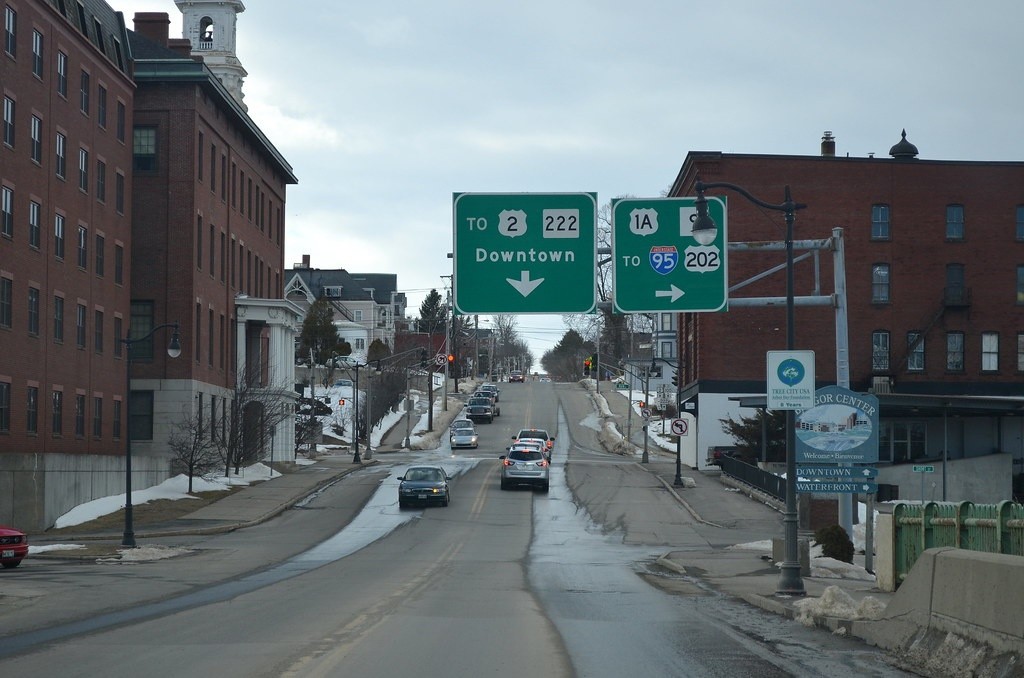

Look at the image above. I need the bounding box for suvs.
[499,429,555,492]
[509,370,524,383]
[464,384,500,423]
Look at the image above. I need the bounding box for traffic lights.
[421,349,428,367]
[448,354,454,362]
[640,402,645,407]
[583,360,590,376]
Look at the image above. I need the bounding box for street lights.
[120,321,182,550]
[692,178,807,596]
[352,359,382,464]
[475,320,489,375]
[649,355,684,486]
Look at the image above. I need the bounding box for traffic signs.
[451,192,597,315]
[611,197,729,314]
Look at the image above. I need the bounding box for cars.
[396,467,452,508]
[325,356,368,369]
[0,525,29,568]
[449,419,479,449]
[540,377,551,382]
